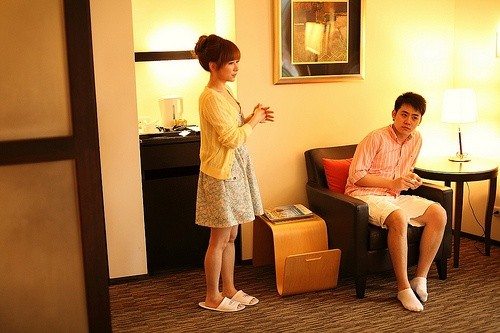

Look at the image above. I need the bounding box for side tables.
[413,157,498,269]
[252,210,342,296]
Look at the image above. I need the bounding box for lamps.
[439,90,477,164]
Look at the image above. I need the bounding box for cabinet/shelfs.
[140,136,215,273]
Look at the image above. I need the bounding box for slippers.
[198,297,245,312]
[219,290,259,306]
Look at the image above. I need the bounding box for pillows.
[322,157,354,194]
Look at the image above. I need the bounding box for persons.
[344,91,447,312]
[191,34,274,312]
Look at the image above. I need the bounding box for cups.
[175,119,187,130]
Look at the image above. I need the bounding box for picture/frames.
[271,0,368,85]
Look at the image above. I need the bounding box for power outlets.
[494,206,500,217]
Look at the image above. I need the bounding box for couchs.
[304,144,453,301]
